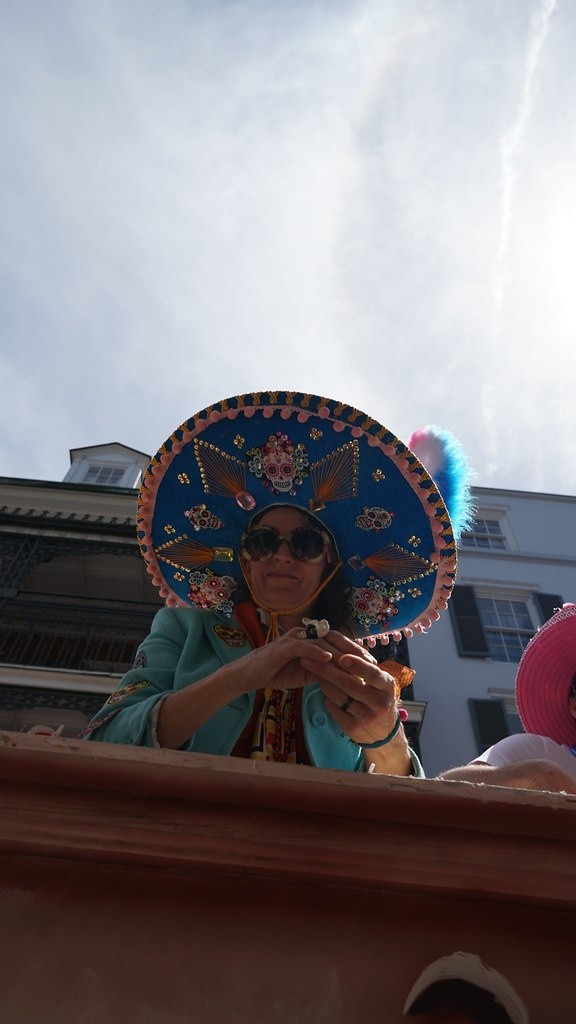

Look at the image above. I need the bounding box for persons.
[437,602,576,794]
[76,391,478,779]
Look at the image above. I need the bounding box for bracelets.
[349,709,409,749]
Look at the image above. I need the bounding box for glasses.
[239,524,331,564]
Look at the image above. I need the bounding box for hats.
[135,391,478,647]
[515,604,576,748]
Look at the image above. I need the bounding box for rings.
[341,696,353,711]
[296,618,330,639]
